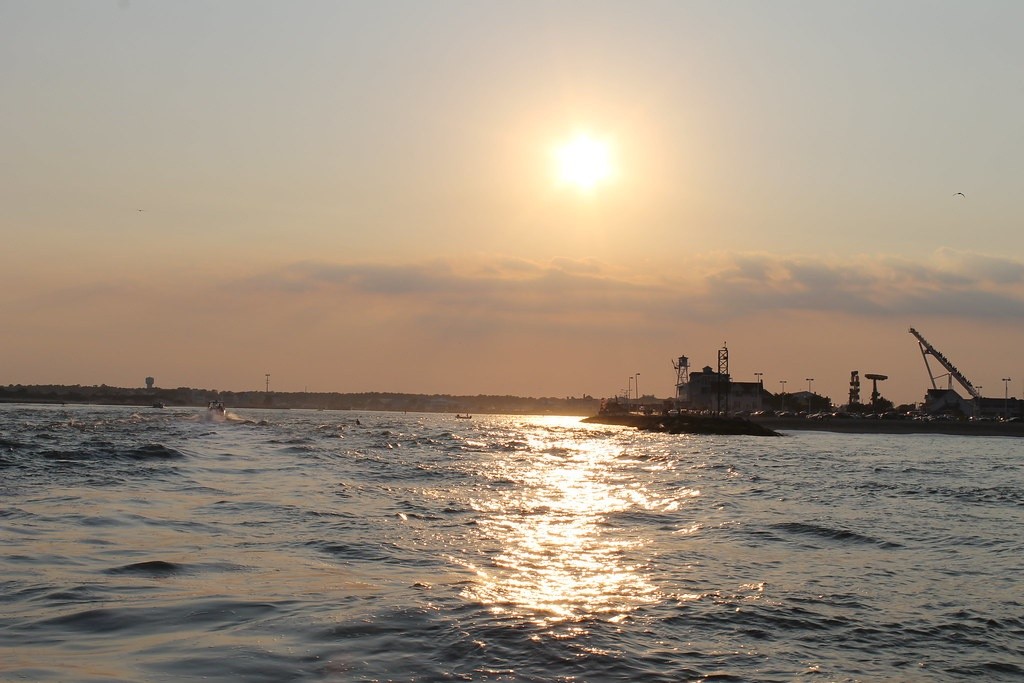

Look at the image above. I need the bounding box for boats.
[208,399,226,417]
[152,400,166,409]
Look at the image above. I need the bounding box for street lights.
[753,372,763,412]
[635,373,641,415]
[779,380,787,413]
[975,385,983,421]
[806,378,815,415]
[629,376,634,414]
[1002,378,1012,420]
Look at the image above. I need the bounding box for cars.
[666,407,1024,425]
